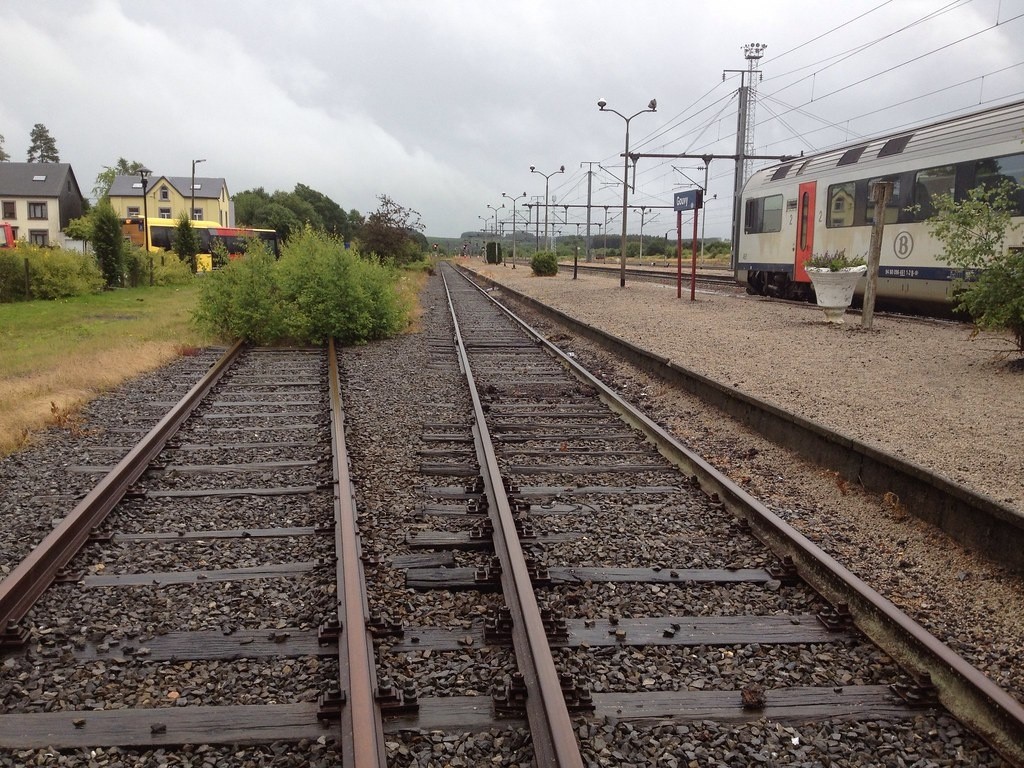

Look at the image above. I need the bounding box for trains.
[733,97,1024,317]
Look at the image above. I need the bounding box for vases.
[804,266,868,323]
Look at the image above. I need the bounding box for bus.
[117,214,280,273]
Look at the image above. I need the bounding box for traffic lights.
[434,243,437,250]
[463,245,466,250]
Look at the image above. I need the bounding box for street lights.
[529,164,565,253]
[135,166,154,254]
[597,97,657,286]
[502,191,527,269]
[478,214,494,261]
[191,158,207,219]
[487,204,505,264]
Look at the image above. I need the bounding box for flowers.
[803,247,870,271]
